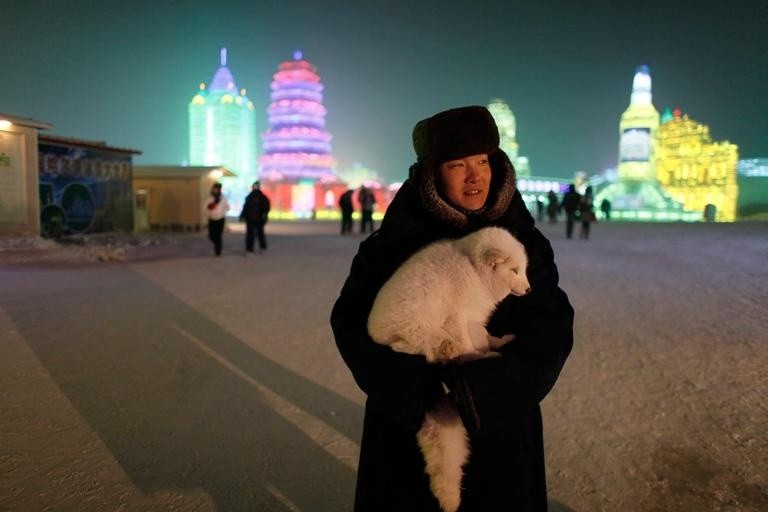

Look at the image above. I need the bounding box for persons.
[338,182,378,236]
[202,182,232,257]
[238,180,272,256]
[535,183,613,243]
[329,149,577,511]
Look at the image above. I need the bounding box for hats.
[409,105,517,227]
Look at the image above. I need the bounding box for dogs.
[365,226,534,512]
